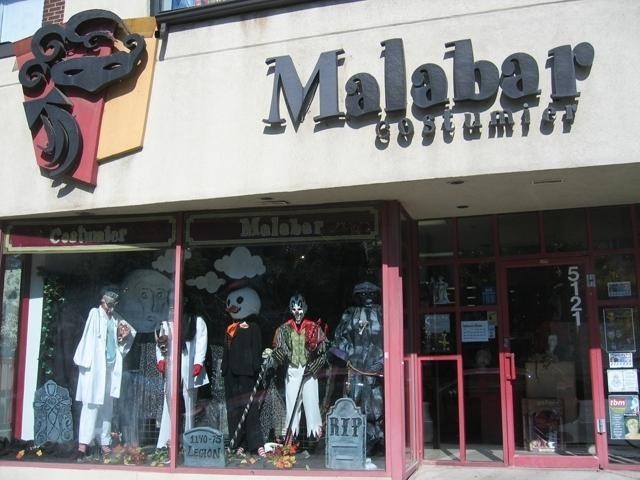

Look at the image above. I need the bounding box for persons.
[261,293,329,452]
[72,285,137,456]
[152,289,208,450]
[326,281,384,458]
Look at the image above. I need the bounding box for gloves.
[157,360,167,373]
[191,364,202,376]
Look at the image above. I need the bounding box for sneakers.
[365,438,385,457]
[294,439,318,454]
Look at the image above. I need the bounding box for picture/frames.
[603,308,636,352]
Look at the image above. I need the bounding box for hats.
[352,281,382,305]
[226,277,248,294]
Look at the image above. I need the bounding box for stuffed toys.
[220,280,263,464]
